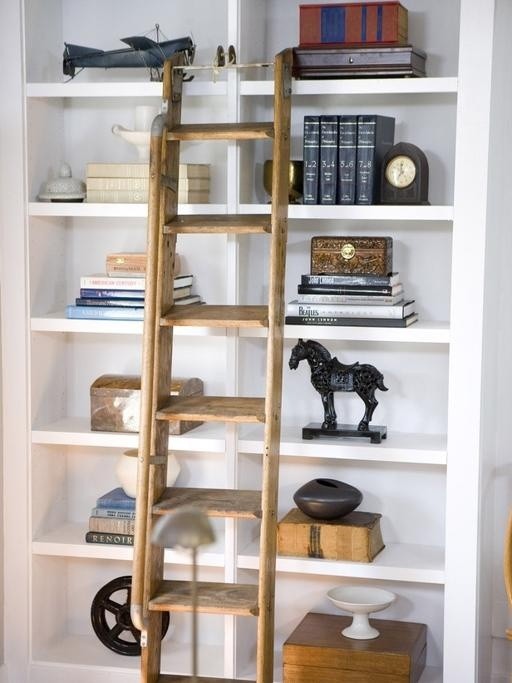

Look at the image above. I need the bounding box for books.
[65,252,206,323]
[284,114,421,330]
[85,487,136,547]
[86,163,211,205]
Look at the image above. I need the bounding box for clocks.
[377,142,430,206]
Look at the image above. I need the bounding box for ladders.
[130,48,293,683]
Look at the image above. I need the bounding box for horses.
[289,339,388,431]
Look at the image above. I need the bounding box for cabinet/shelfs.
[1,1,512,683]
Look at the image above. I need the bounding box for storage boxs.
[283,612,426,683]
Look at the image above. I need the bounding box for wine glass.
[325,586,396,640]
